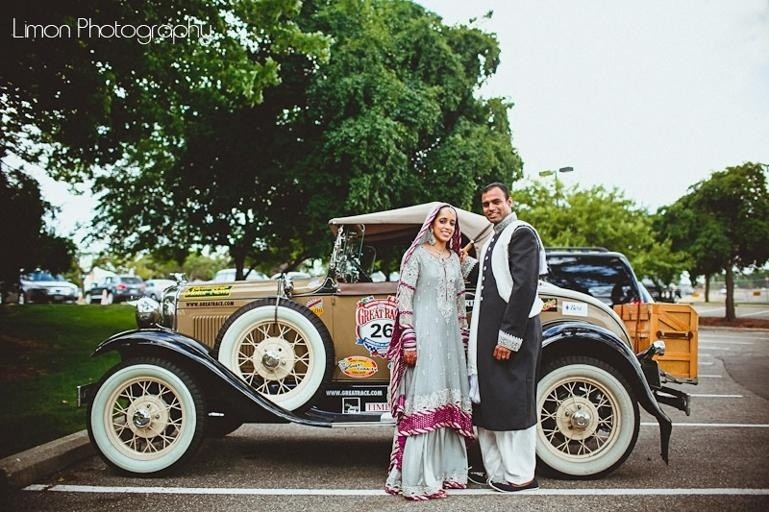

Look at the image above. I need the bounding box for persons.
[381,201,474,503]
[456,182,549,495]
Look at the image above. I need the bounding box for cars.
[538,245,690,317]
[0,262,270,305]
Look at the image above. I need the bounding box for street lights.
[538,166,574,205]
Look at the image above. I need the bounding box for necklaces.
[430,242,449,259]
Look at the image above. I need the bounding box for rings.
[501,355,506,359]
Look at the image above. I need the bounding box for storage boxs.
[612,303,699,385]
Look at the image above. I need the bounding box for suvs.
[68,201,690,481]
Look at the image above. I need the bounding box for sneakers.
[468,472,488,485]
[489,479,539,492]
